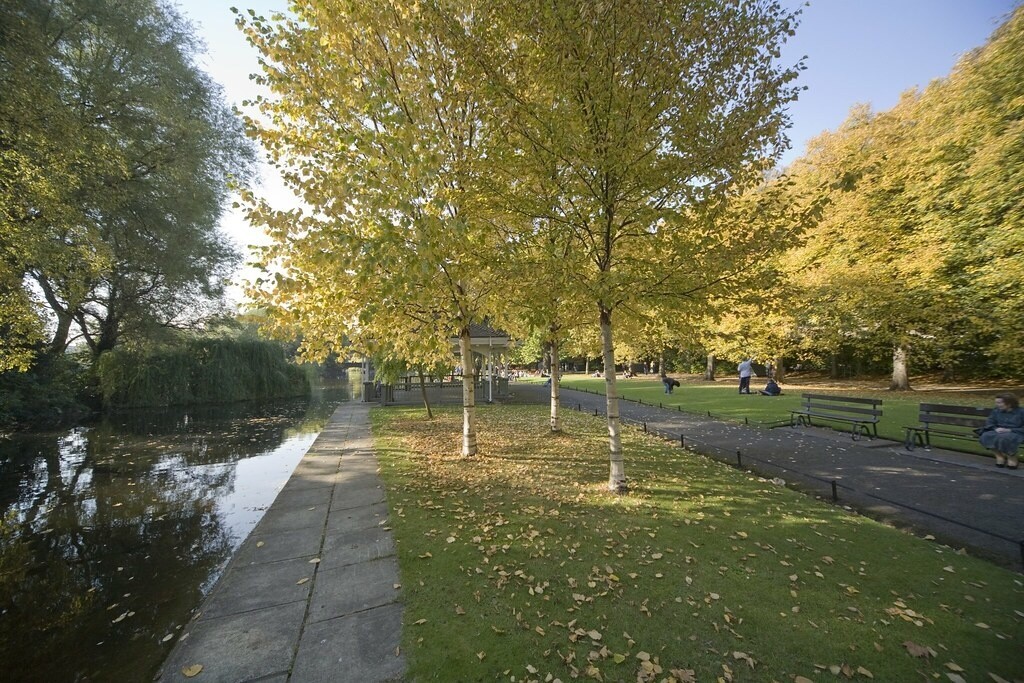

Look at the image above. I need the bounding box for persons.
[758,379,781,396]
[644,361,648,375]
[662,378,680,395]
[623,370,637,379]
[592,370,601,377]
[973,393,1024,468]
[737,353,757,394]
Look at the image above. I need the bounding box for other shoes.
[747,392,752,394]
[739,392,744,394]
[1007,461,1019,469]
[996,458,1005,467]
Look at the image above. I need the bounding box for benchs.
[786,393,883,441]
[549,375,562,387]
[902,403,1024,452]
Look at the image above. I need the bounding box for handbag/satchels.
[972,428,984,437]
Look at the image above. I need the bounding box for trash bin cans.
[497,376,509,395]
[380,384,394,402]
[363,382,377,402]
